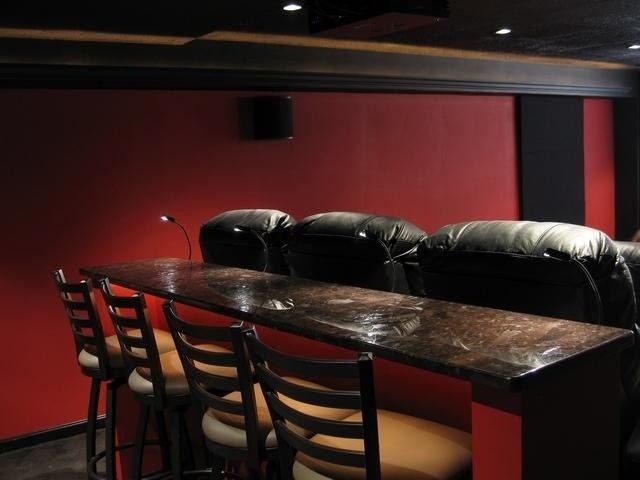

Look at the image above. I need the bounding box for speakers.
[237,96,294,140]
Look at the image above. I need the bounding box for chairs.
[98,279,266,478]
[162,301,359,478]
[199,208,291,275]
[53,269,185,479]
[417,220,638,325]
[288,212,427,297]
[243,328,473,477]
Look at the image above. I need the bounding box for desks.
[80,260,639,478]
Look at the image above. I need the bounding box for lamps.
[160,215,192,260]
